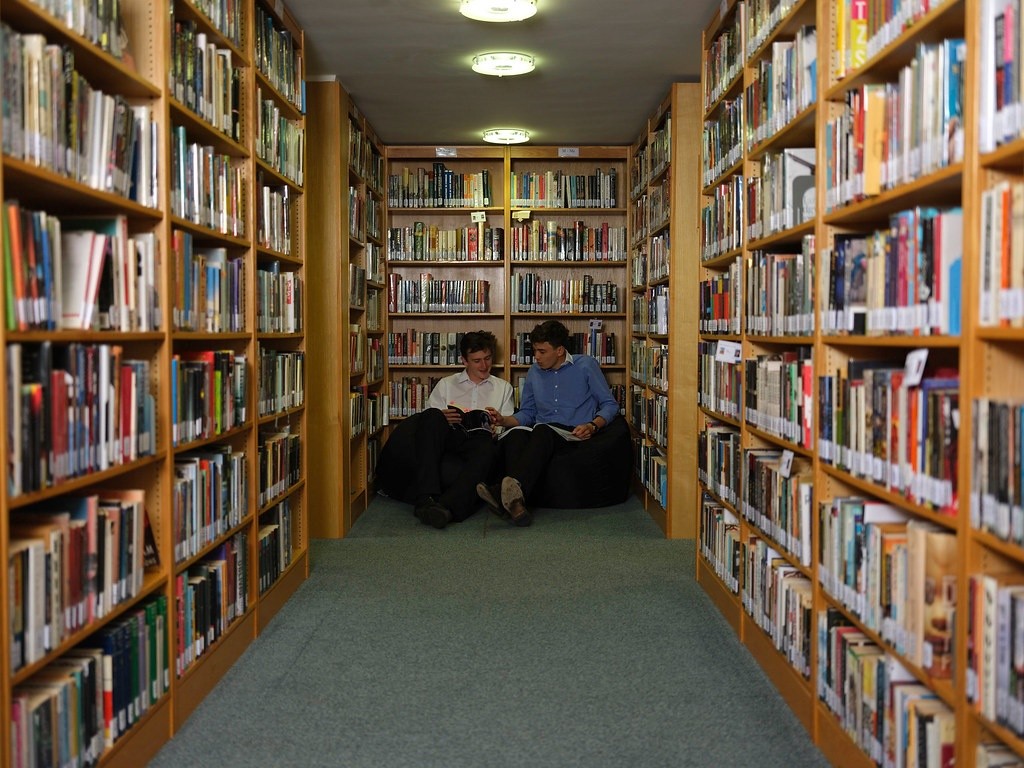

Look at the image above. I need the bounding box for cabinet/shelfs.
[0,0,309,768]
[385,144,507,443]
[309,74,385,540]
[630,82,701,540]
[694,0,1024,768]
[506,144,630,423]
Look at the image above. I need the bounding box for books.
[447,405,493,430]
[0,0,1024,768]
[456,424,492,438]
[498,423,582,441]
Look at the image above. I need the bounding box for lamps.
[471,52,537,77]
[481,128,532,146]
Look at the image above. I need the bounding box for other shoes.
[414,505,450,529]
[501,476,531,527]
[476,482,500,510]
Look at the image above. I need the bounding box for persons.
[414,330,514,529]
[475,320,620,527]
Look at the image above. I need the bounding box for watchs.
[588,422,600,432]
